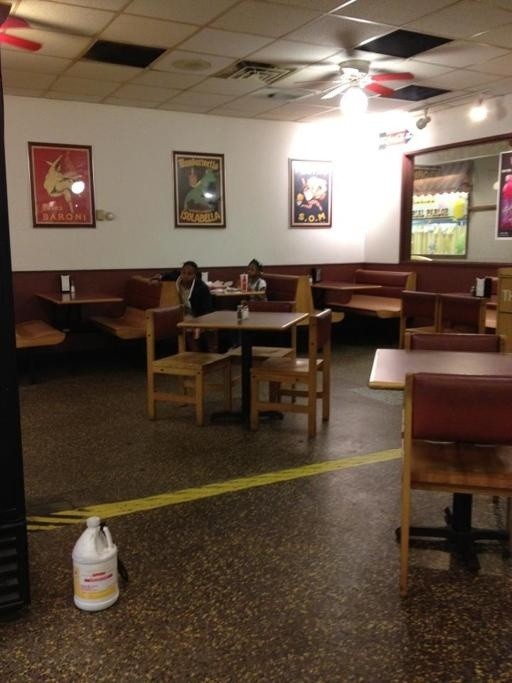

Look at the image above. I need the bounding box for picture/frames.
[495,150,512,241]
[27,141,96,228]
[290,158,333,228]
[172,150,226,229]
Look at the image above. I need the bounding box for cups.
[240,274,248,291]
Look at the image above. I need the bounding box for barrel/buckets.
[71,517,121,613]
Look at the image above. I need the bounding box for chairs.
[399,290,500,352]
[249,308,331,438]
[225,300,297,404]
[399,371,511,596]
[145,304,231,427]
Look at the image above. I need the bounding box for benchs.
[14,318,66,375]
[259,269,417,354]
[87,275,181,371]
[485,275,499,329]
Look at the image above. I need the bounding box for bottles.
[484,277,491,298]
[236,304,249,320]
[71,280,76,292]
[303,271,312,284]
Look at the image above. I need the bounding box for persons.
[183,168,212,211]
[244,259,268,302]
[174,260,214,319]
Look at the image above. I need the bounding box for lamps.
[339,77,368,112]
[416,109,430,129]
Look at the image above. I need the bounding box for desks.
[210,287,265,310]
[368,348,512,572]
[177,310,310,432]
[35,290,125,371]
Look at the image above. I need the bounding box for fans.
[295,59,414,101]
[0,0,42,52]
